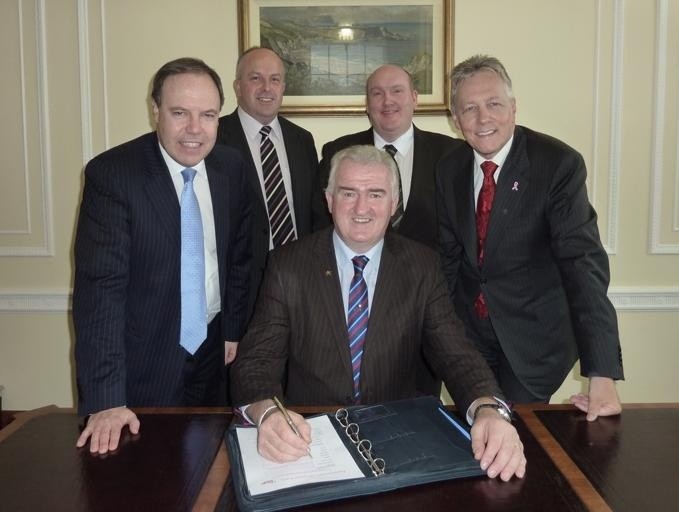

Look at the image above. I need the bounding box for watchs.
[472,401,512,422]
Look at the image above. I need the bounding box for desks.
[0,400,677,512]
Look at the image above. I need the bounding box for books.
[224,393,492,510]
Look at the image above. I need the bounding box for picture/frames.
[234,1,457,120]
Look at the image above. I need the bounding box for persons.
[321,65,470,404]
[436,57,626,423]
[231,143,528,484]
[75,57,255,455]
[219,46,325,308]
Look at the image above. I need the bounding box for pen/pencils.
[272,395,316,462]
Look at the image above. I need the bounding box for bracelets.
[257,404,277,429]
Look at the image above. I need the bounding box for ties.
[383,144,404,230]
[179,168,207,356]
[259,126,296,249]
[476,161,498,319]
[348,254,370,404]
